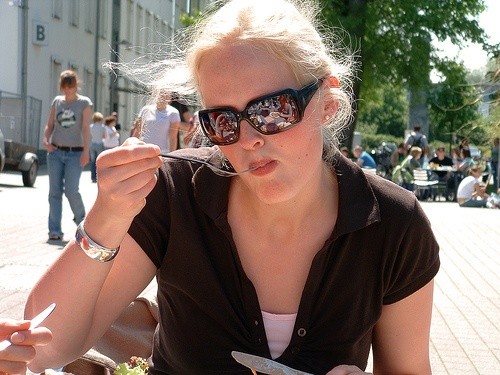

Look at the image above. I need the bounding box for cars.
[0,143,41,187]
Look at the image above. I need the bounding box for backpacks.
[410,133,423,148]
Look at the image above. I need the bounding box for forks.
[159,154,260,176]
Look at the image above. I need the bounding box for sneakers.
[48,235,61,245]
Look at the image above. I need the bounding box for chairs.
[412,167,439,201]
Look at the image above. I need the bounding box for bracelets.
[75,220,120,262]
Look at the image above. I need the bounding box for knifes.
[231,350,312,375]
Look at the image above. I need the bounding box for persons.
[102,112,121,149]
[41,69,94,244]
[257,94,292,120]
[428,147,453,203]
[352,146,376,168]
[399,147,423,184]
[340,146,348,157]
[451,137,500,209]
[129,84,182,155]
[89,112,106,183]
[168,88,200,149]
[25,0,440,375]
[0,319,54,375]
[404,125,430,164]
[391,142,407,168]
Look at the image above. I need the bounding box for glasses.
[199,78,325,146]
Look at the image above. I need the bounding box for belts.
[51,143,84,152]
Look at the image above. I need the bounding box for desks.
[426,164,462,201]
